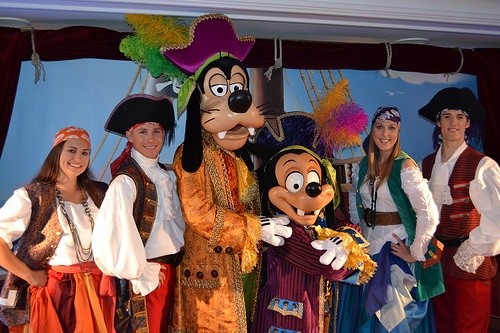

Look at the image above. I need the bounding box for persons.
[419,105,500,333]
[106,118,186,333]
[0,126,118,333]
[348,105,439,332]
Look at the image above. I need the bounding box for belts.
[156,251,186,266]
[362,206,403,227]
[438,234,472,248]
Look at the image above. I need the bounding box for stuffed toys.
[160,13,293,333]
[253,110,364,333]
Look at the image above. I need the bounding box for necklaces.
[369,170,381,230]
[55,183,95,276]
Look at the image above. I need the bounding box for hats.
[104,94,175,137]
[418,87,485,133]
